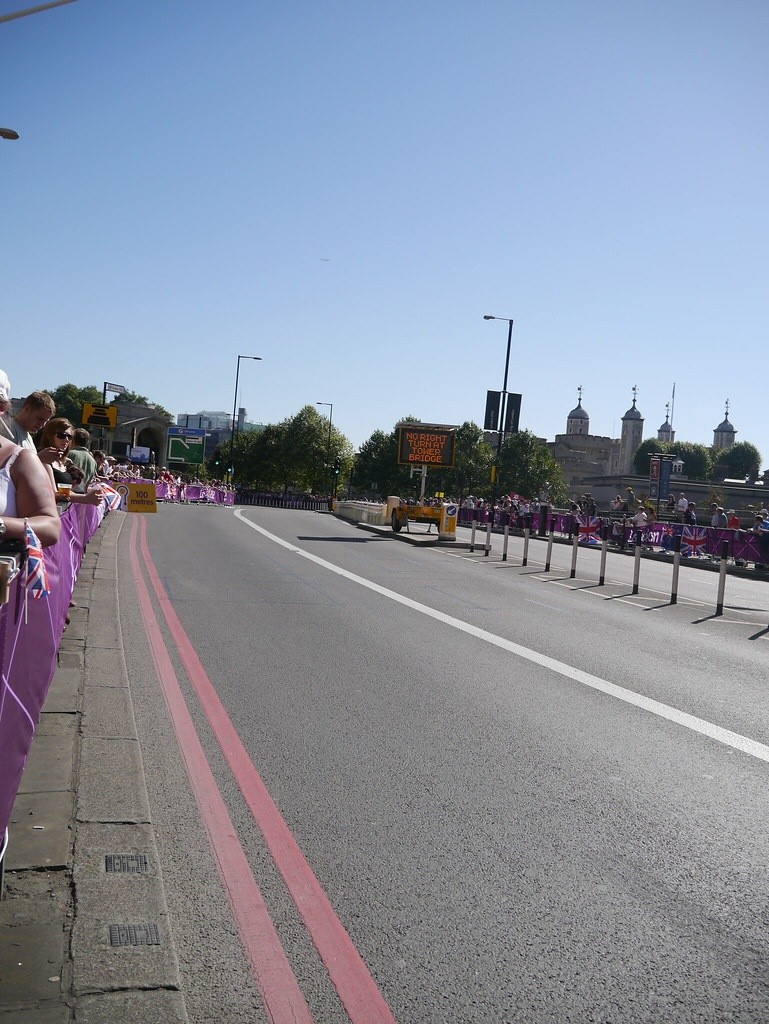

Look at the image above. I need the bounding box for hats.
[759,509,769,514]
[124,458,132,461]
[105,456,117,461]
[727,509,735,514]
[716,507,724,511]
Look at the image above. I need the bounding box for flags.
[96,483,123,512]
[24,516,51,599]
[576,515,710,559]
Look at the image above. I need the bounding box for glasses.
[71,474,82,484]
[52,432,74,441]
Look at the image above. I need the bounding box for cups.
[96,491,103,503]
[58,483,72,497]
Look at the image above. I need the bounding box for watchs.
[0,517,7,537]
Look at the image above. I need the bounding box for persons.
[0,369,236,551]
[400,487,769,533]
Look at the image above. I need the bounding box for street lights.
[483,315,514,529]
[226,355,262,485]
[317,402,332,491]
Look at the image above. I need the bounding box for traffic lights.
[226,461,232,473]
[334,457,340,476]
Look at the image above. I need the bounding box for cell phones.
[57,449,65,453]
